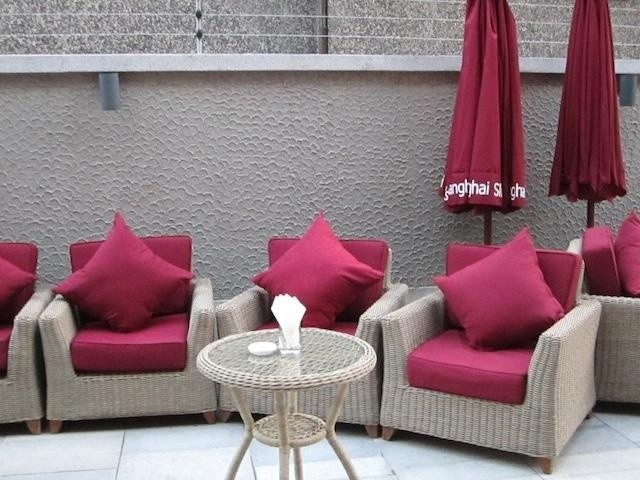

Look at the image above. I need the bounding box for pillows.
[250,211,383,336]
[616,210,639,298]
[52,209,194,337]
[429,228,565,350]
[0,254,34,299]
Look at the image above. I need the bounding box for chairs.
[218,236,411,443]
[561,225,640,411]
[0,235,51,439]
[382,248,604,475]
[37,236,220,432]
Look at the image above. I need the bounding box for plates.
[247,341,278,358]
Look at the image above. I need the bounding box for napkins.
[270,292,309,345]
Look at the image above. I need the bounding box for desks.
[195,327,377,478]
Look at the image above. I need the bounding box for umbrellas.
[545,0,627,237]
[439,0,527,244]
